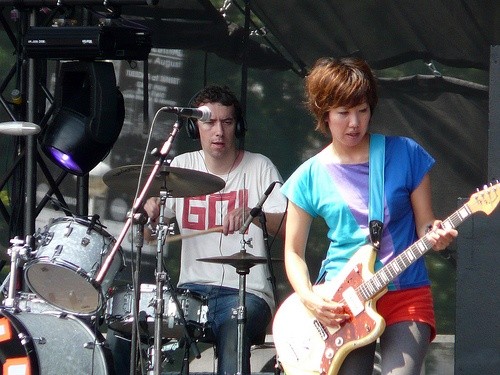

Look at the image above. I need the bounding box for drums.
[105,284,209,342]
[23,216,126,316]
[0,305,107,375]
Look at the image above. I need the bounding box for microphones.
[161,106,211,122]
[239,181,275,234]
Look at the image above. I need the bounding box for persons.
[279,57,458,375]
[144,87,287,375]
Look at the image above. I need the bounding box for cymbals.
[196,257,281,266]
[102,164,226,197]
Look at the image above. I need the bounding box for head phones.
[185,90,247,140]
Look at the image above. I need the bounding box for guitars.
[272,181,500,375]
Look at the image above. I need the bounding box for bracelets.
[425,224,432,235]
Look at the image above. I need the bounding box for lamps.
[39,62,126,175]
